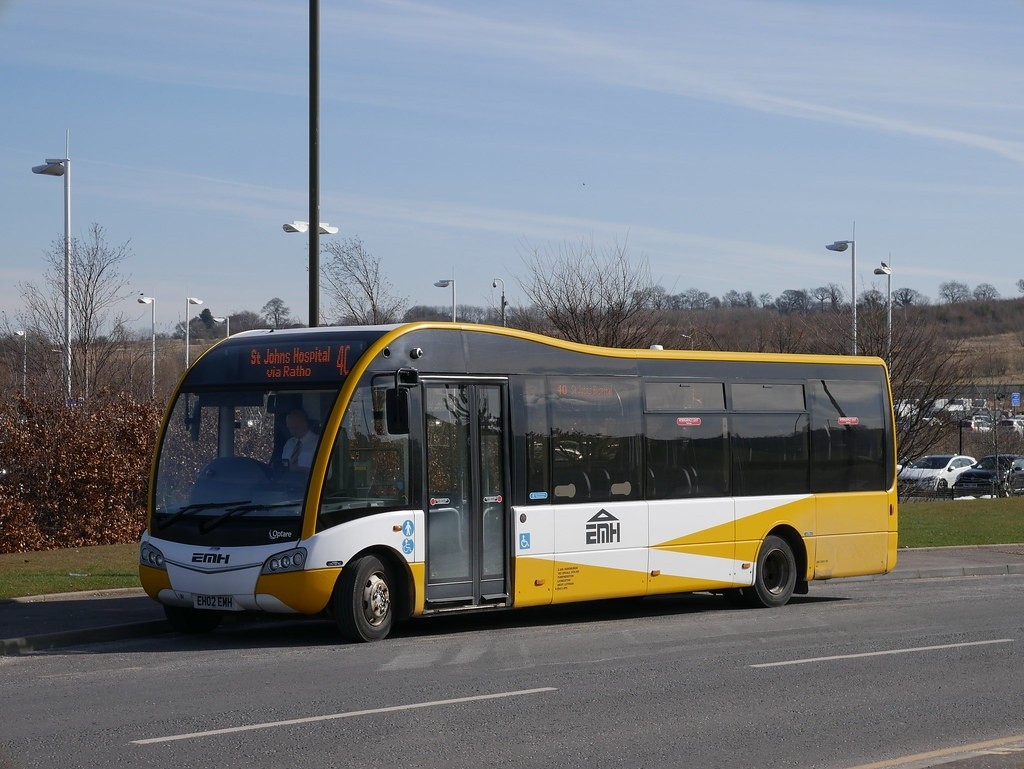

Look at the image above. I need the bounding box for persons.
[280,410,332,494]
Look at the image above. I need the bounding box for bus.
[139,319,899,642]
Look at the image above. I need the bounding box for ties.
[291,439,300,464]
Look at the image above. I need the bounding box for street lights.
[434,279,457,322]
[825,220,857,356]
[14,331,27,396]
[31,127,73,398]
[216,316,229,337]
[185,297,204,417]
[874,251,893,383]
[137,297,156,397]
[492,277,507,328]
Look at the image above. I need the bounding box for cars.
[897,454,983,497]
[951,453,1024,499]
[893,397,1024,444]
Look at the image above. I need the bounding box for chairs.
[518,460,881,505]
[313,426,353,497]
